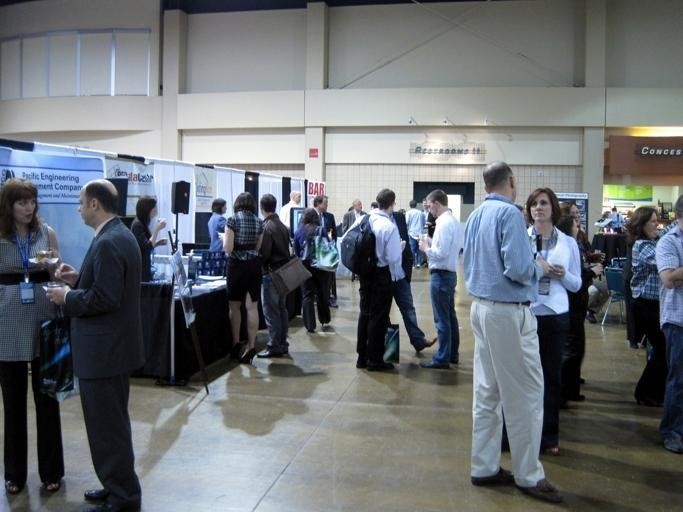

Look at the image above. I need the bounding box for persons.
[207,187,462,374]
[462,161,565,504]
[501,186,683,462]
[130,197,167,283]
[0,176,63,493]
[42,178,145,512]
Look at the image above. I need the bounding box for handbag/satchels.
[39,317,80,404]
[269,255,313,297]
[297,235,316,261]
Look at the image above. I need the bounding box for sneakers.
[662,432,683,453]
[472,467,515,486]
[514,479,563,503]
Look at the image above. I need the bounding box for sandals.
[5,479,23,494]
[44,480,61,491]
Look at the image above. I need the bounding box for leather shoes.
[84,502,140,512]
[84,488,110,499]
[546,446,561,456]
[586,311,597,323]
[229,294,462,369]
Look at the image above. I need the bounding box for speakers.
[171,180,190,214]
[106,177,128,217]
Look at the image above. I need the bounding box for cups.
[37,249,52,266]
[420,229,429,248]
[157,217,167,230]
[48,281,67,303]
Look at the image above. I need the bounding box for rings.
[557,272,561,276]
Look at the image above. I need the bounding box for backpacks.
[341,214,378,275]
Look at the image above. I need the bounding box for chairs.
[601,267,626,326]
[611,257,628,272]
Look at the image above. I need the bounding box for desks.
[592,232,628,264]
[168,274,227,382]
